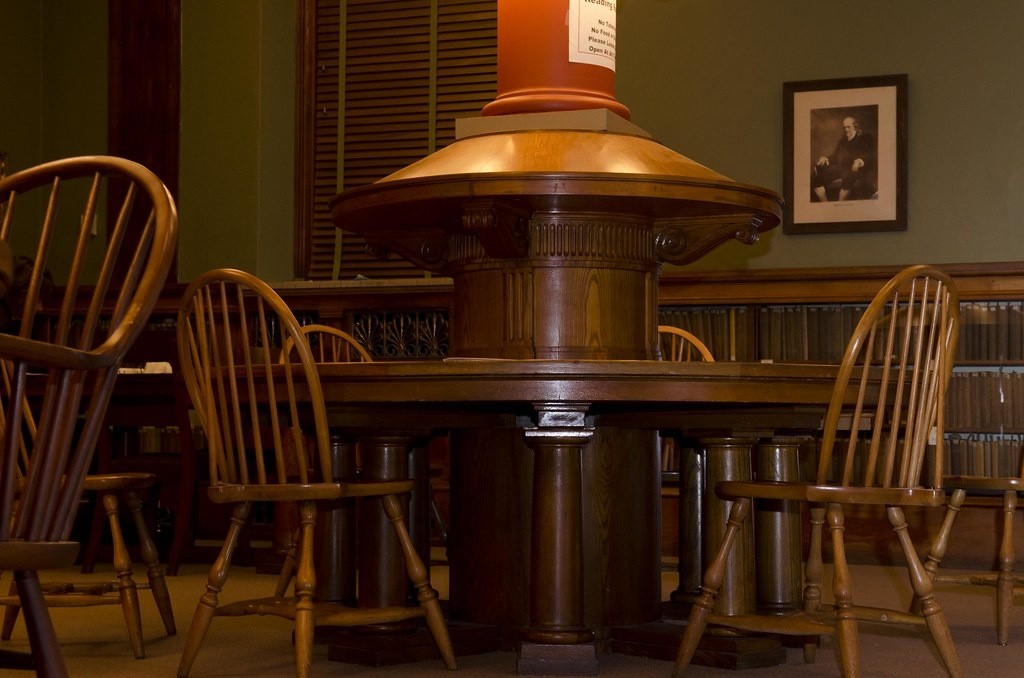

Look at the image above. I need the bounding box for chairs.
[0,356,177,658]
[0,156,178,678]
[672,266,966,678]
[274,324,376,598]
[908,474,1024,646]
[177,268,460,678]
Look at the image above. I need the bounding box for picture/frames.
[781,73,908,235]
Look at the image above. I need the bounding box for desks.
[201,359,923,678]
[7,371,193,573]
